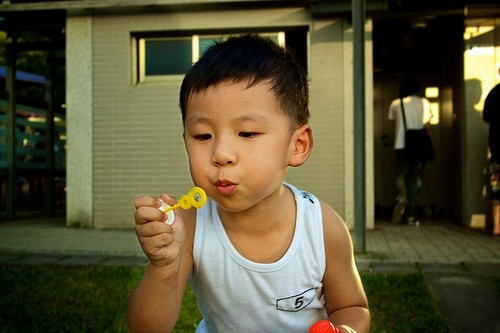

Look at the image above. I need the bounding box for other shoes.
[408,217,419,225]
[393,200,407,224]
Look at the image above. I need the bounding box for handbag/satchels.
[400,98,436,163]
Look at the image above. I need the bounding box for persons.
[387,80,441,225]
[482,83,500,171]
[126,35,370,333]
